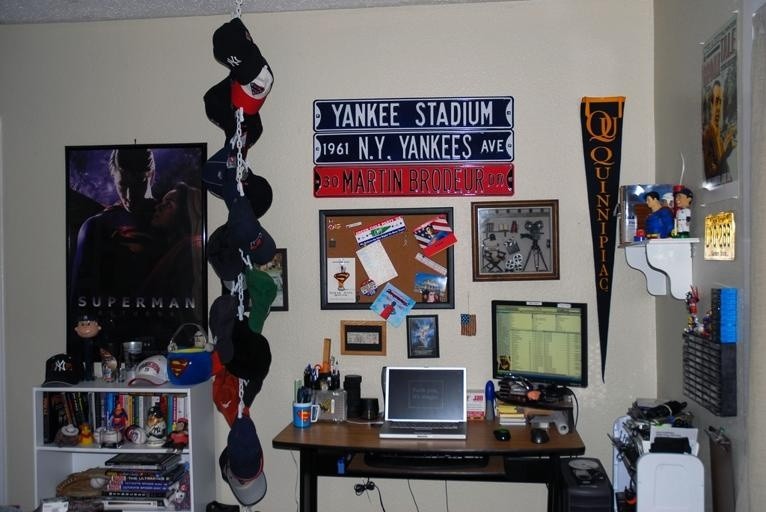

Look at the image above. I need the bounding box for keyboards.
[363,450,490,470]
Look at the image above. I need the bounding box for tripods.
[523,240,548,272]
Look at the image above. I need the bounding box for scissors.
[314,361,330,372]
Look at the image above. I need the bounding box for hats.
[203,74,263,148]
[204,351,241,427]
[226,327,272,409]
[217,448,269,506]
[212,17,265,85]
[207,223,245,283]
[246,267,279,334]
[209,293,238,364]
[126,352,171,387]
[41,352,80,388]
[204,141,274,220]
[226,414,263,477]
[226,197,276,265]
[229,64,275,116]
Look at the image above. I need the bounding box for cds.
[569,459,599,470]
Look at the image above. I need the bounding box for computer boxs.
[559,457,613,512]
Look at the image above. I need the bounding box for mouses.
[530,428,549,444]
[493,428,511,441]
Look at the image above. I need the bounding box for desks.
[270,416,587,512]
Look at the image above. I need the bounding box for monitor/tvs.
[491,300,587,410]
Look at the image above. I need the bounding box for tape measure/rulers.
[323,339,331,372]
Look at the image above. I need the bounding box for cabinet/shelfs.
[31,373,218,512]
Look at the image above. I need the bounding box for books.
[496,401,527,426]
[104,453,188,509]
[42,391,186,448]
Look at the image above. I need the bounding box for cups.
[292,402,321,428]
[123,342,143,369]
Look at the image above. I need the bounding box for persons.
[73,313,103,381]
[424,226,444,244]
[74,145,198,329]
[673,183,694,237]
[382,301,396,318]
[644,192,674,239]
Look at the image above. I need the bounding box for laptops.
[378,367,467,440]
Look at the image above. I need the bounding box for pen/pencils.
[294,360,319,403]
[329,356,339,375]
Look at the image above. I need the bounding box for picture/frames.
[338,318,388,358]
[405,313,441,360]
[317,205,455,312]
[62,142,209,358]
[258,247,290,313]
[469,199,560,282]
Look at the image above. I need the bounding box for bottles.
[361,398,379,419]
[101,351,115,381]
[343,374,362,418]
[145,404,166,447]
